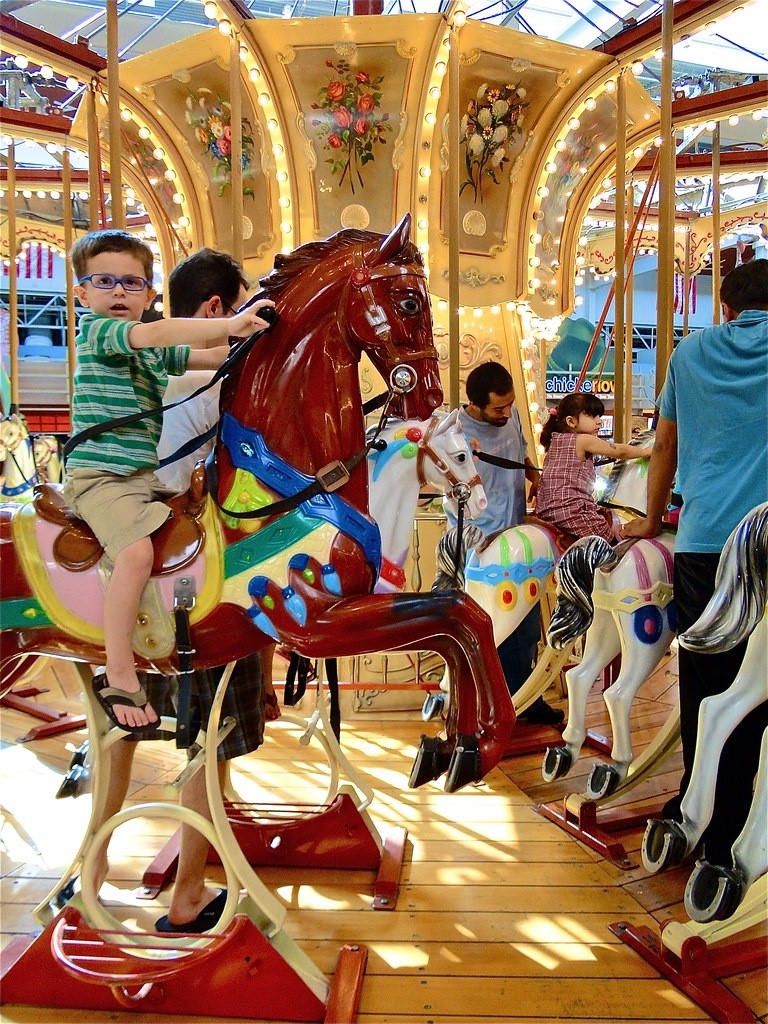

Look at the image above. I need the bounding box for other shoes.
[518,699,564,725]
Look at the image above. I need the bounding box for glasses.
[78,273,153,291]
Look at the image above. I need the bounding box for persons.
[623,259,768,865]
[62,228,276,734]
[56,246,265,938]
[441,362,565,725]
[535,392,653,546]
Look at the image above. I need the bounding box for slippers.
[154,888,229,934]
[93,672,161,733]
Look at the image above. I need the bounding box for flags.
[3,241,52,279]
[673,273,697,315]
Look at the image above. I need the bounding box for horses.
[1,213,768,929]
